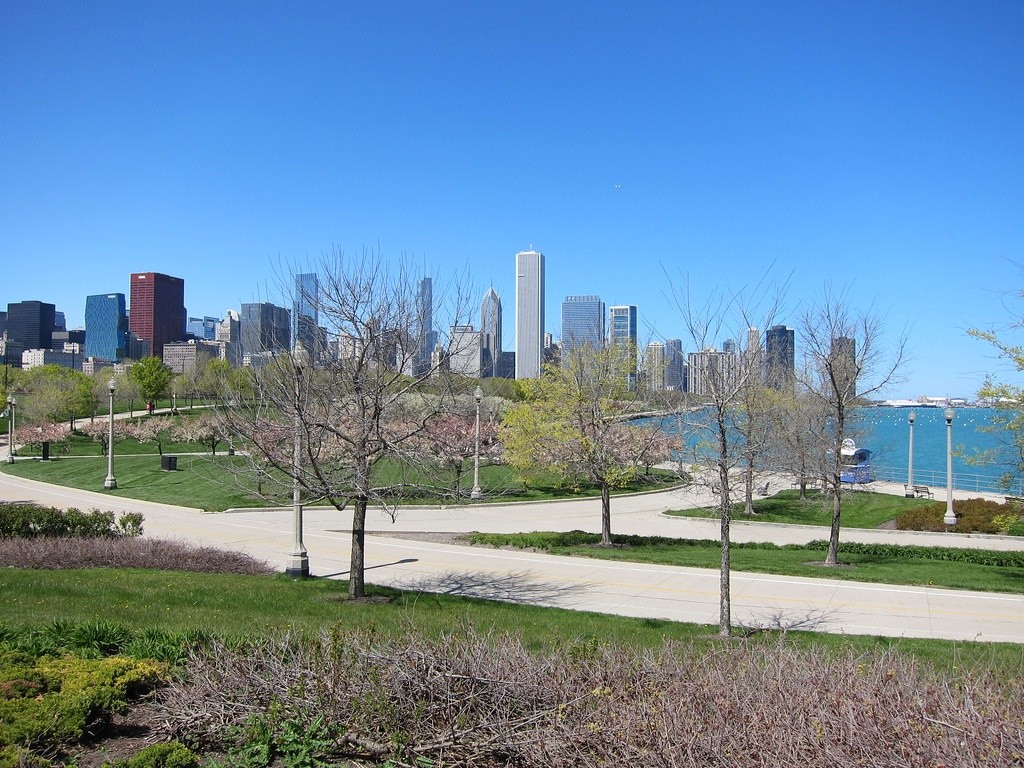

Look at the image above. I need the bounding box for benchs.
[1004,496,1024,504]
[904,484,934,500]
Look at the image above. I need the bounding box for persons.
[146,401,155,414]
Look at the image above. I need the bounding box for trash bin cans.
[161,455,178,469]
[835,438,874,484]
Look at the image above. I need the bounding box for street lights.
[944,409,957,525]
[905,411,916,498]
[470,386,483,499]
[228,400,235,456]
[11,398,17,456]
[6,393,15,465]
[286,343,309,577]
[676,405,685,480]
[173,379,177,411]
[104,377,119,489]
